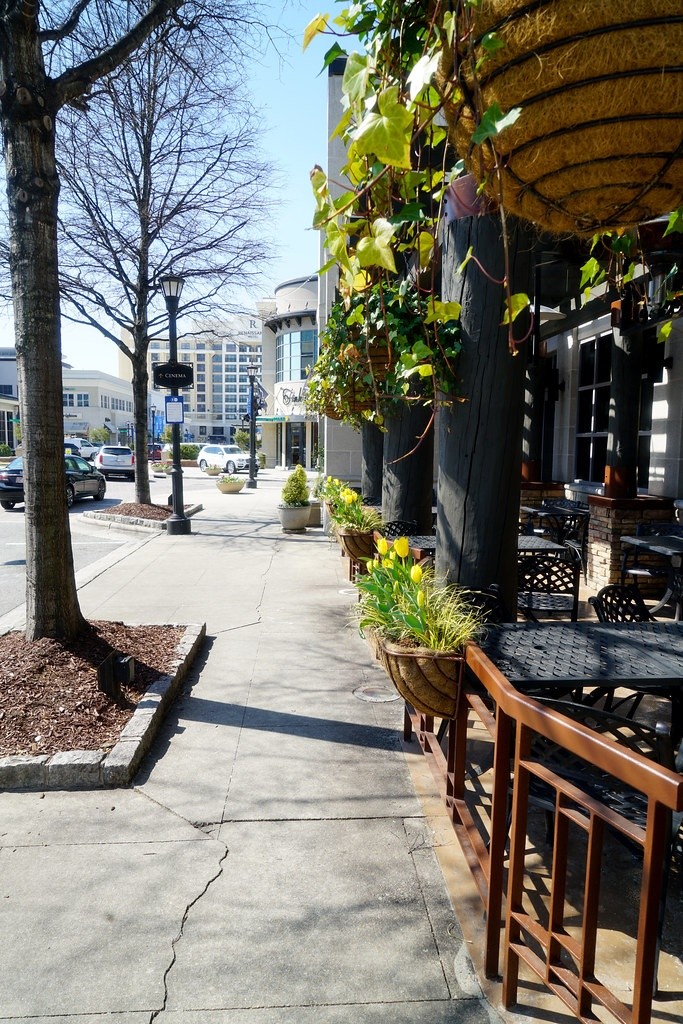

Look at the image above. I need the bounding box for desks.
[385,535,568,623]
[618,535,683,621]
[479,621,683,774]
[364,505,437,527]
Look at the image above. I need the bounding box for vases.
[372,627,463,720]
[336,524,373,564]
[325,501,334,518]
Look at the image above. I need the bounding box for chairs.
[365,494,683,998]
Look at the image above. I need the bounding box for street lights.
[246,364,259,488]
[156,265,196,535]
[150,403,156,463]
[126,419,131,447]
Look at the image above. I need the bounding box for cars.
[147,444,161,461]
[0,455,107,509]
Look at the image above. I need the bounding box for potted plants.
[204,464,222,476]
[277,463,312,534]
[302,0,683,468]
[150,463,169,471]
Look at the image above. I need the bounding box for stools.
[307,500,321,525]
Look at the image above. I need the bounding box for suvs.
[64,437,100,462]
[93,445,135,482]
[197,445,259,474]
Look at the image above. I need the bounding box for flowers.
[339,536,507,662]
[322,475,351,500]
[333,488,390,532]
